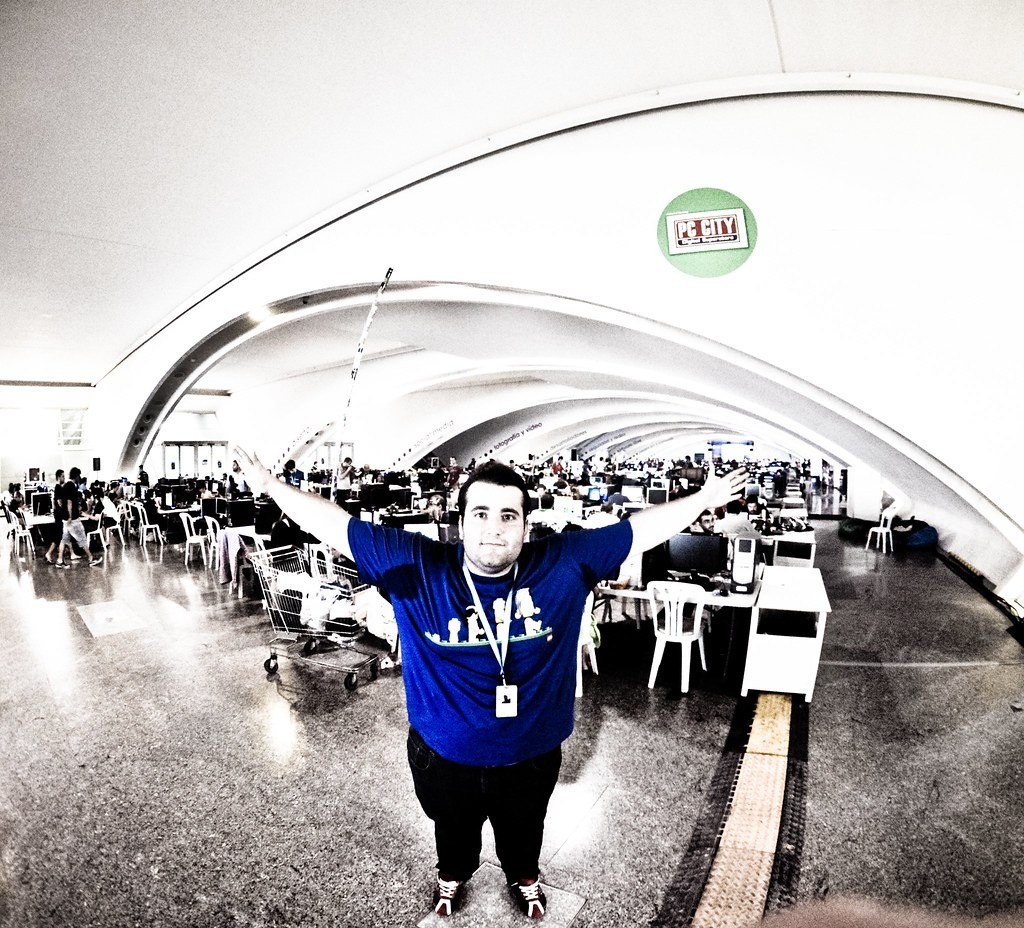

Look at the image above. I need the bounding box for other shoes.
[101,544,110,549]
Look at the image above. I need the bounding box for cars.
[765,461,785,472]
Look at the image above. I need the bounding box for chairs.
[1,501,766,696]
[865,507,899,553]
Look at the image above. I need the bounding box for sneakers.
[507,878,547,919]
[432,873,465,917]
[55,560,70,570]
[88,554,103,567]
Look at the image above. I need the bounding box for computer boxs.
[730,536,762,594]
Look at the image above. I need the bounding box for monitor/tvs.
[20,467,461,546]
[528,468,773,593]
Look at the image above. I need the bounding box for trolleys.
[244,544,382,689]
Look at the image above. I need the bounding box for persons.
[43,469,82,565]
[232,446,750,920]
[2,460,247,542]
[54,468,102,570]
[277,457,848,585]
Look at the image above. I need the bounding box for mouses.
[719,589,728,596]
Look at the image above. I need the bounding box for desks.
[20,484,833,704]
